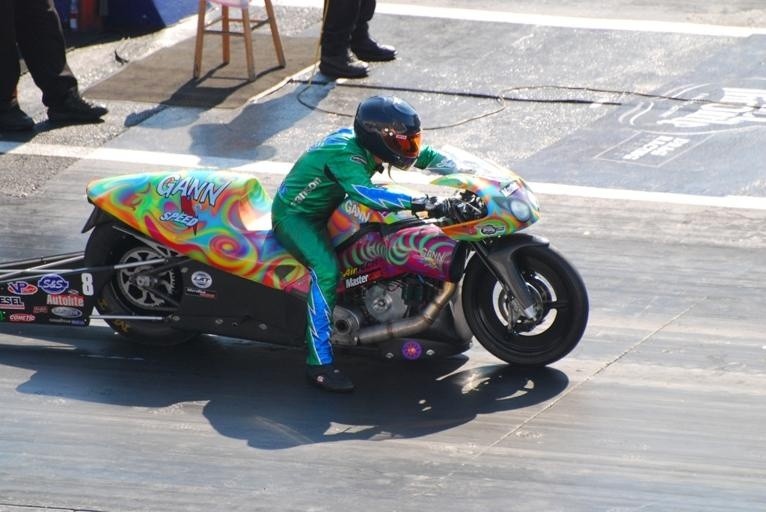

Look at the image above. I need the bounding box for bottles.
[68,1,79,33]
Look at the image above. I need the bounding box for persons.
[271,95,479,392]
[0,0,109,132]
[318,0,397,78]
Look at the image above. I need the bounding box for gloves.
[411,196,450,219]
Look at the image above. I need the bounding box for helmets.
[353,95,420,170]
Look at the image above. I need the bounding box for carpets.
[84,28,334,109]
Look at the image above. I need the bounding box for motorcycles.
[81,155,588,372]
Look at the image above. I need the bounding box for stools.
[194,0,286,80]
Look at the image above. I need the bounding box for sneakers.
[318,54,368,77]
[46,97,107,121]
[0,110,33,130]
[305,365,354,392]
[351,36,395,61]
